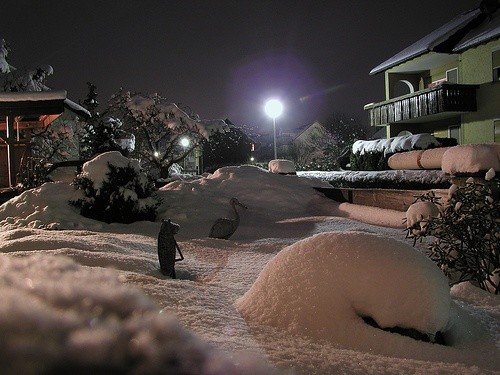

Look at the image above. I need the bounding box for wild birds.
[208,197,249,240]
